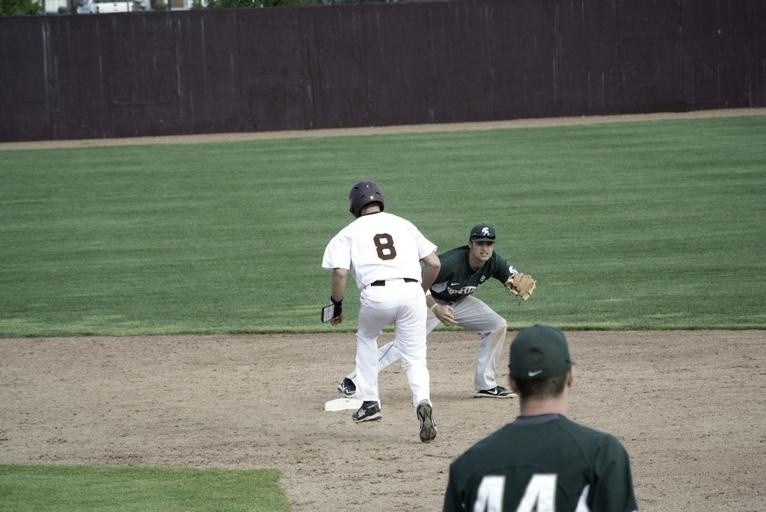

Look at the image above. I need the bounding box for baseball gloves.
[505,273,536,301]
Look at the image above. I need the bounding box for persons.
[321,181,441,444]
[337,222,536,400]
[442,325,639,512]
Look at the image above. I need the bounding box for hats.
[469,224,496,243]
[509,324,575,380]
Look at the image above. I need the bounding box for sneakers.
[352,401,383,423]
[337,378,356,398]
[418,401,437,444]
[474,385,519,398]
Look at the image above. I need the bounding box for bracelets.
[430,303,438,311]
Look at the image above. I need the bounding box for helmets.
[349,180,384,217]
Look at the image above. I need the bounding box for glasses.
[472,231,495,239]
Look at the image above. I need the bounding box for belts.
[370,278,417,286]
